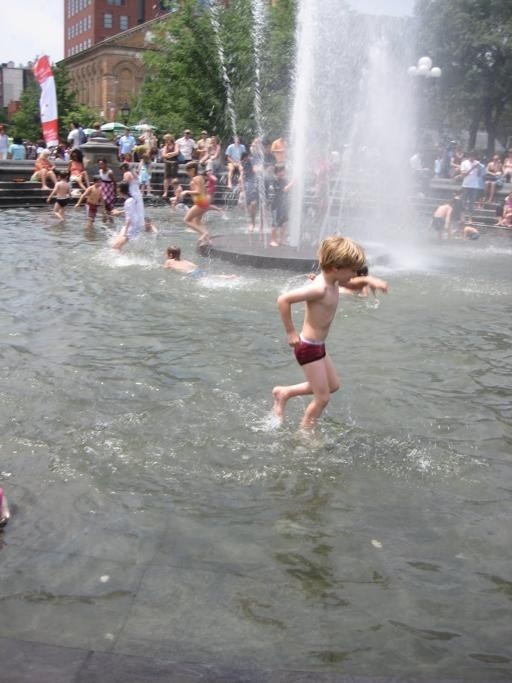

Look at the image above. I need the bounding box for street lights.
[408,56,443,155]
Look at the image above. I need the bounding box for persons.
[309,266,369,297]
[161,245,207,278]
[0,487,10,527]
[2,121,511,253]
[272,236,389,431]
